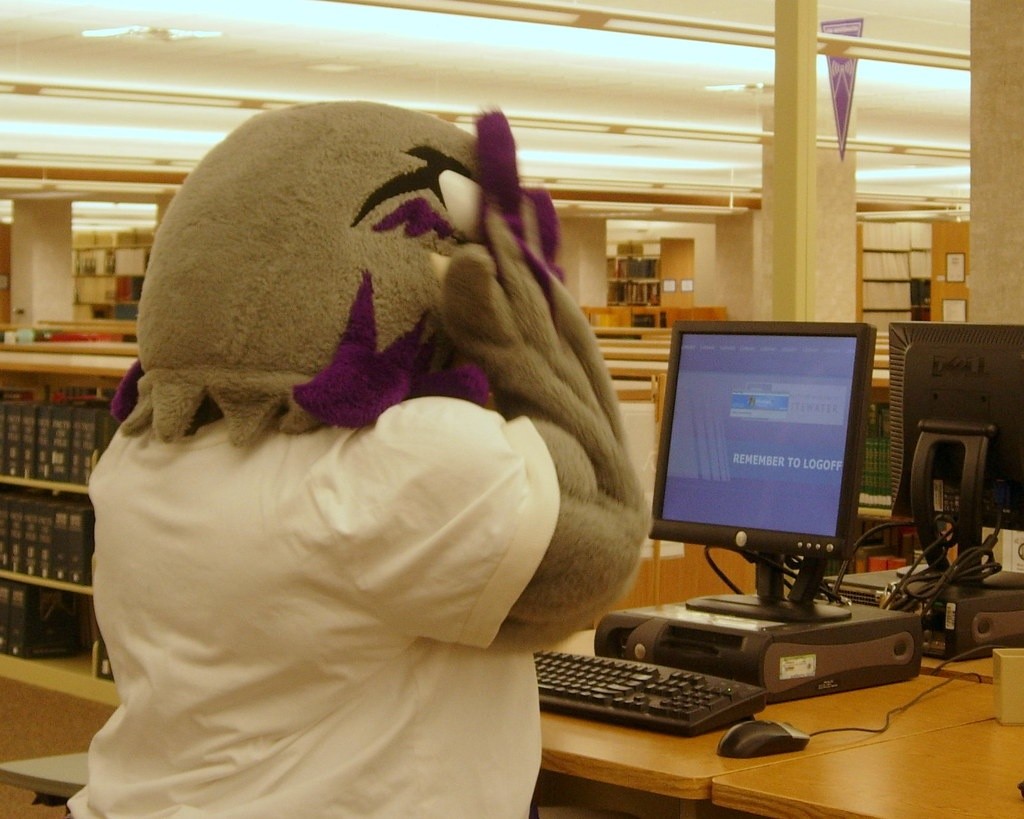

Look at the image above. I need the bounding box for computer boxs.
[821,569,1024,660]
[595,600,924,706]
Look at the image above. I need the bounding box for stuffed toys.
[64,102,647,819]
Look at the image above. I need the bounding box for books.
[910,279,932,323]
[824,401,915,578]
[1,387,131,681]
[69,235,154,322]
[604,259,658,305]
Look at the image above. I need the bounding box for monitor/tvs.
[887,322,1024,589]
[647,321,877,621]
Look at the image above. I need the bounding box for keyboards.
[533,650,768,737]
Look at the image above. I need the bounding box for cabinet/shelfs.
[0,349,143,708]
[577,237,755,631]
[854,218,971,391]
[70,244,152,308]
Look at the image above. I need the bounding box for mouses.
[717,720,810,759]
